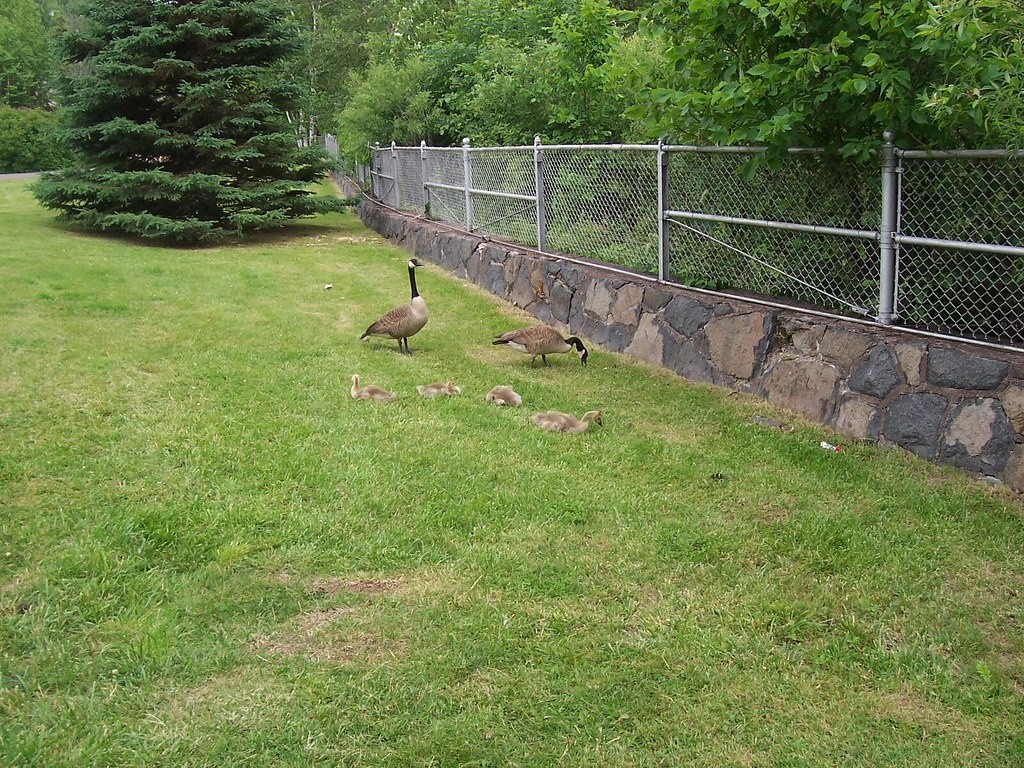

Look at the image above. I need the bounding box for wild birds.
[349,374,396,401]
[360,259,428,353]
[485,385,523,407]
[416,381,462,396]
[529,411,603,435]
[491,326,588,368]
